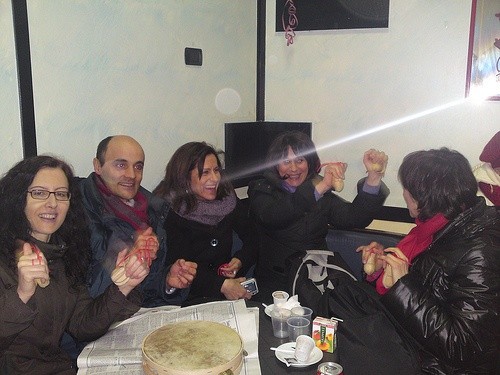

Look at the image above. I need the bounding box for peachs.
[313,330,334,353]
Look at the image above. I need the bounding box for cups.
[272,291,289,306]
[294,335,315,362]
[271,307,313,342]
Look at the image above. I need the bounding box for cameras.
[240,278,259,295]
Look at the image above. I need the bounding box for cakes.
[142,320,243,375]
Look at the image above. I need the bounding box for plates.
[264,304,305,318]
[275,342,323,367]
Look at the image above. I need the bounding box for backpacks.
[288,248,362,318]
[314,277,422,375]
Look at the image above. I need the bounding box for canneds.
[316,362,344,375]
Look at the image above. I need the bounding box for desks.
[179,295,339,375]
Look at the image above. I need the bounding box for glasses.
[27,189,71,201]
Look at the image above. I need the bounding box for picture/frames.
[463,0,500,101]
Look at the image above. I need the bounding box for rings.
[32,258,35,265]
[140,256,145,261]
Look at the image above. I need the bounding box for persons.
[149,141,255,301]
[0,156,150,375]
[249,130,392,295]
[471,130,500,208]
[65,135,198,321]
[356,147,500,375]
